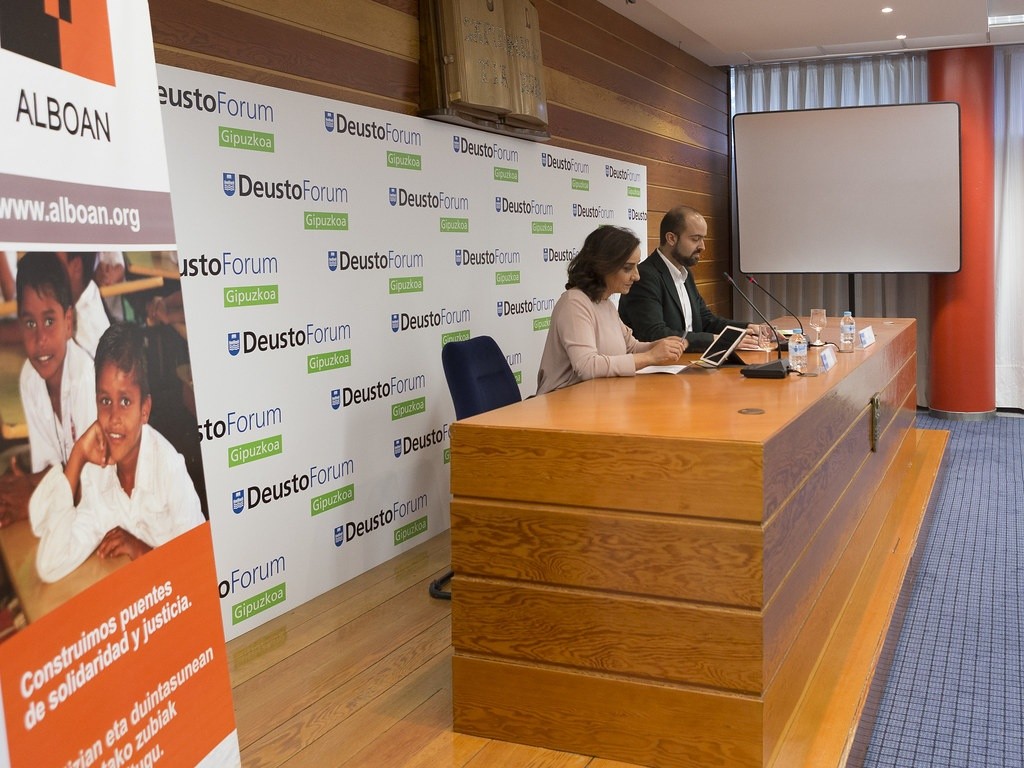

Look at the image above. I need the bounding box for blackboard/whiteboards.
[732,101,961,274]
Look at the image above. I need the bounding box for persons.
[618,205,787,350]
[0,251,208,586]
[536,223,689,396]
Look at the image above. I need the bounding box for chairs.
[426,335,538,601]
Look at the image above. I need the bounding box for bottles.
[788,329,807,376]
[840,311,856,353]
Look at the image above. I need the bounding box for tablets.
[700,326,747,366]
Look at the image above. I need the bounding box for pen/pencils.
[680,325,691,344]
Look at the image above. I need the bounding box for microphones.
[723,271,812,378]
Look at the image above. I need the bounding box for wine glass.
[758,325,778,362]
[810,308,827,346]
